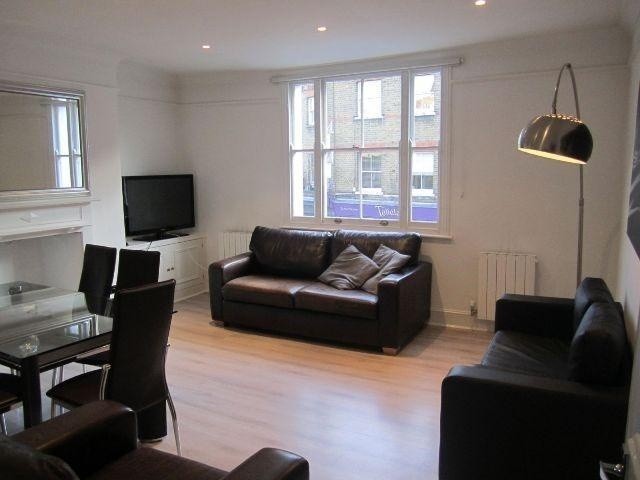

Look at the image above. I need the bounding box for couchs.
[0,399,309,480]
[209,225,432,356]
[439,277,634,480]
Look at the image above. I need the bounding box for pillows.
[360,243,411,295]
[318,244,380,290]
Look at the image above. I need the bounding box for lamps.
[517,63,593,286]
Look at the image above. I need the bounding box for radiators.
[477,251,536,321]
[217,230,252,258]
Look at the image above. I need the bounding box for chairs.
[60,248,161,422]
[46,279,181,456]
[0,372,27,436]
[51,244,117,418]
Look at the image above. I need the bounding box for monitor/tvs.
[122,173,196,242]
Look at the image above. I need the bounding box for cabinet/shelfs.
[126,230,208,303]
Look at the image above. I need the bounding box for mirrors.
[0,78,93,209]
